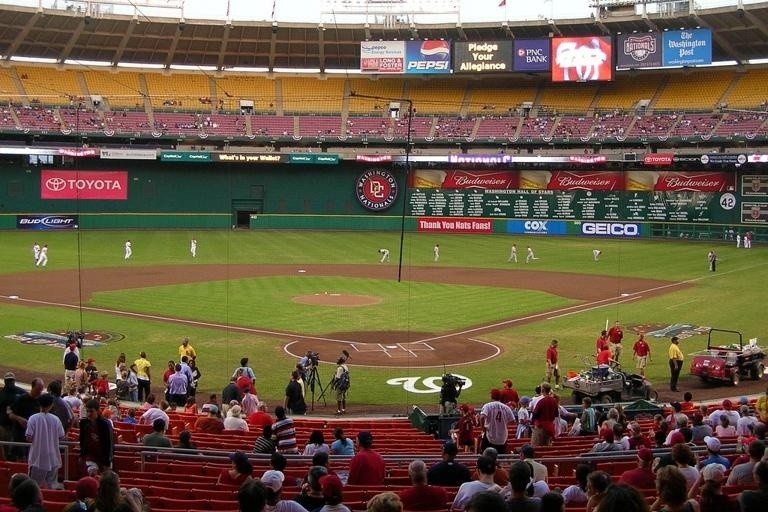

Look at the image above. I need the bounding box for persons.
[709,251,717,272]
[377,249,391,265]
[705,250,712,271]
[507,243,520,263]
[0,321,766,510]
[189,236,197,258]
[32,242,40,259]
[35,244,48,268]
[432,244,440,262]
[735,232,752,249]
[525,246,540,264]
[123,240,133,261]
[592,248,602,262]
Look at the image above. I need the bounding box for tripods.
[307,366,326,411]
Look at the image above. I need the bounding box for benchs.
[0,399,768,512]
[0,107,768,138]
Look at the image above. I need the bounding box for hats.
[319,474,342,497]
[503,380,512,385]
[670,401,681,409]
[506,397,532,407]
[704,436,720,451]
[638,448,652,461]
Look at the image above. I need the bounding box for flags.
[498,0,507,7]
